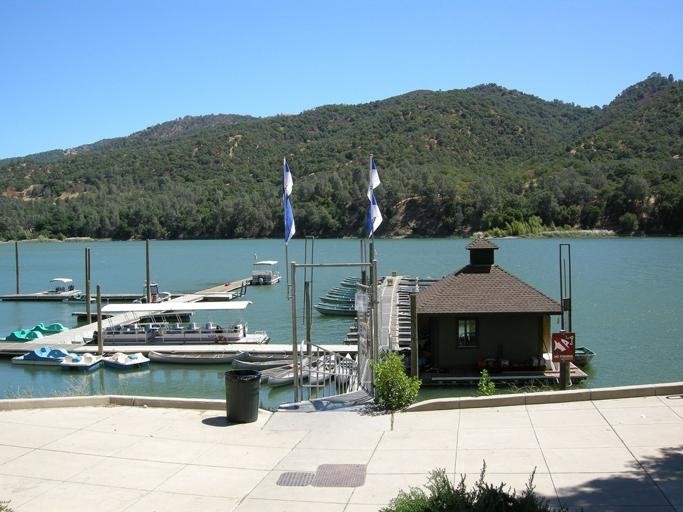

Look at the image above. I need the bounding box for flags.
[366,156,382,240]
[282,158,296,244]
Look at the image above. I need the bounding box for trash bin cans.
[224,370,263,423]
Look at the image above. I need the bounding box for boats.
[312,275,360,316]
[249,252,282,286]
[36,277,82,295]
[573,345,596,366]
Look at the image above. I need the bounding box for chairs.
[118,323,218,332]
